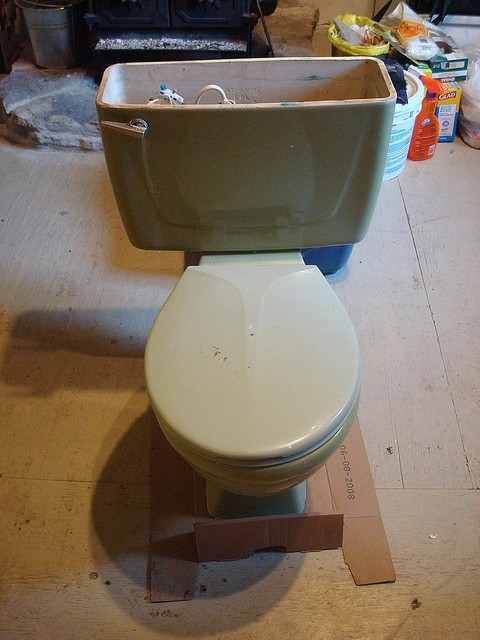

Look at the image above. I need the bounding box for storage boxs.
[430,35,468,82]
[424,81,462,141]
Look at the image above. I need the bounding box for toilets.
[95,57,396,514]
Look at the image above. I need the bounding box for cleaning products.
[409,74,441,160]
